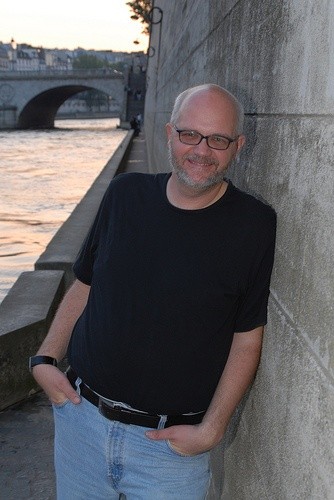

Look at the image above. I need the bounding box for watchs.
[28,355,58,373]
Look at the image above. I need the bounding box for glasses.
[173,123,239,150]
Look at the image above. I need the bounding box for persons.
[126,86,145,137]
[28,83,277,500]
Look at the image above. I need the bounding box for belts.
[67,369,205,428]
